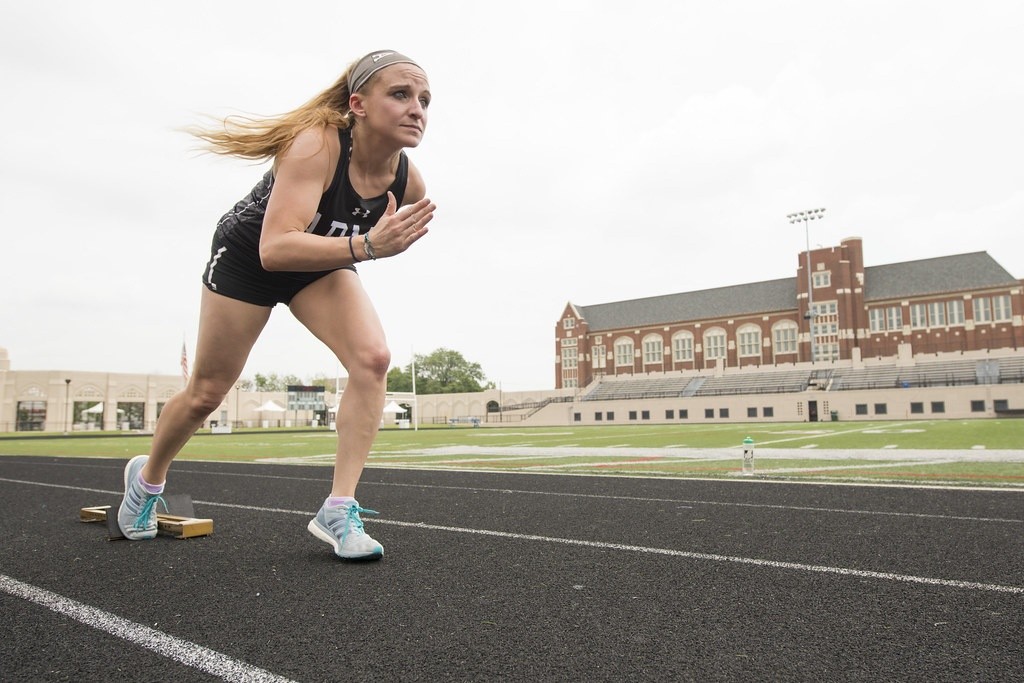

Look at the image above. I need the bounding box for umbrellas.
[383,401,408,413]
[253,399,286,412]
[81,402,125,413]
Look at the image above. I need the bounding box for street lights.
[786,207,826,363]
[63,377,72,433]
[235,385,240,428]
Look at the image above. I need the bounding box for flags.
[180,338,189,382]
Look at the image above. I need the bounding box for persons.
[117,48,436,562]
[348,234,362,263]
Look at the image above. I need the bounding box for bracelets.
[364,231,377,261]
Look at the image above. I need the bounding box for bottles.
[742,437,754,475]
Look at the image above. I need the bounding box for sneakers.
[308,495,384,563]
[117,456,170,541]
